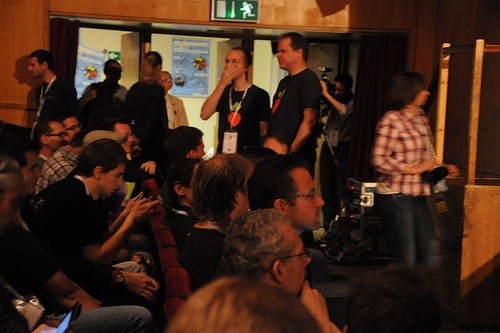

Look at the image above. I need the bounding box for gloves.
[422,166,449,186]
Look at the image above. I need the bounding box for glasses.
[46,131,67,137]
[65,122,83,131]
[272,191,316,206]
[161,78,170,83]
[262,247,310,276]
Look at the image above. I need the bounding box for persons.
[0,32,443,333]
[369,72,460,265]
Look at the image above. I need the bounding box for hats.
[83,129,128,148]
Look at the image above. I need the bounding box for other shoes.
[318,231,339,247]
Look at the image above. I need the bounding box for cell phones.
[137,194,154,201]
[55,301,82,333]
